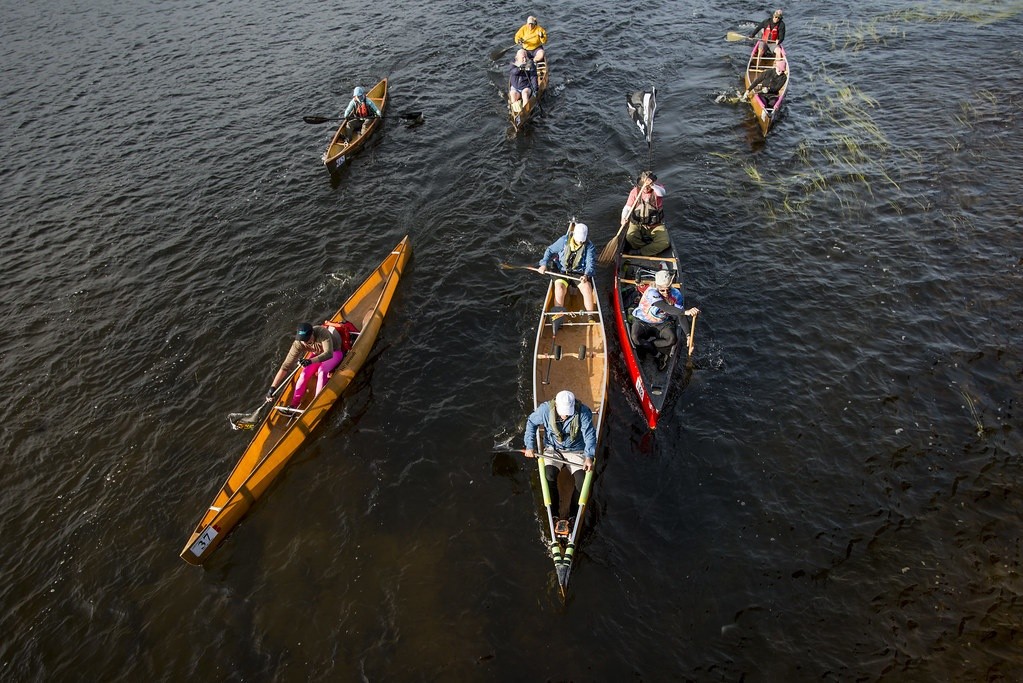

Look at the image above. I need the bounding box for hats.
[295,323,312,341]
[573,223,588,242]
[774,10,782,18]
[556,390,576,415]
[353,87,363,96]
[527,16,535,24]
[777,61,786,71]
[636,171,657,187]
[654,270,675,288]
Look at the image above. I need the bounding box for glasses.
[660,288,671,292]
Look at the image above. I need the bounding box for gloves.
[520,64,526,71]
[533,91,538,98]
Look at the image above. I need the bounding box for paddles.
[497,261,580,281]
[728,90,763,104]
[523,67,545,117]
[490,34,539,61]
[597,183,647,270]
[490,446,584,468]
[727,32,776,42]
[232,365,301,430]
[303,111,422,125]
[686,316,697,368]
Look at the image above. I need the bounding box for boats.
[179,233,413,567]
[745,37,790,138]
[533,279,610,600]
[507,54,550,133]
[614,221,685,432]
[324,77,388,175]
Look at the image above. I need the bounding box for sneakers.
[636,347,644,363]
[655,353,668,371]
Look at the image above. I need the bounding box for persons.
[631,270,700,368]
[538,224,598,328]
[344,87,383,142]
[748,10,785,65]
[524,391,597,531]
[620,171,671,256]
[265,322,343,417]
[509,49,538,107]
[515,15,547,63]
[742,61,786,113]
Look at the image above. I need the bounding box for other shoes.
[345,138,351,142]
[278,409,293,417]
[587,315,595,323]
